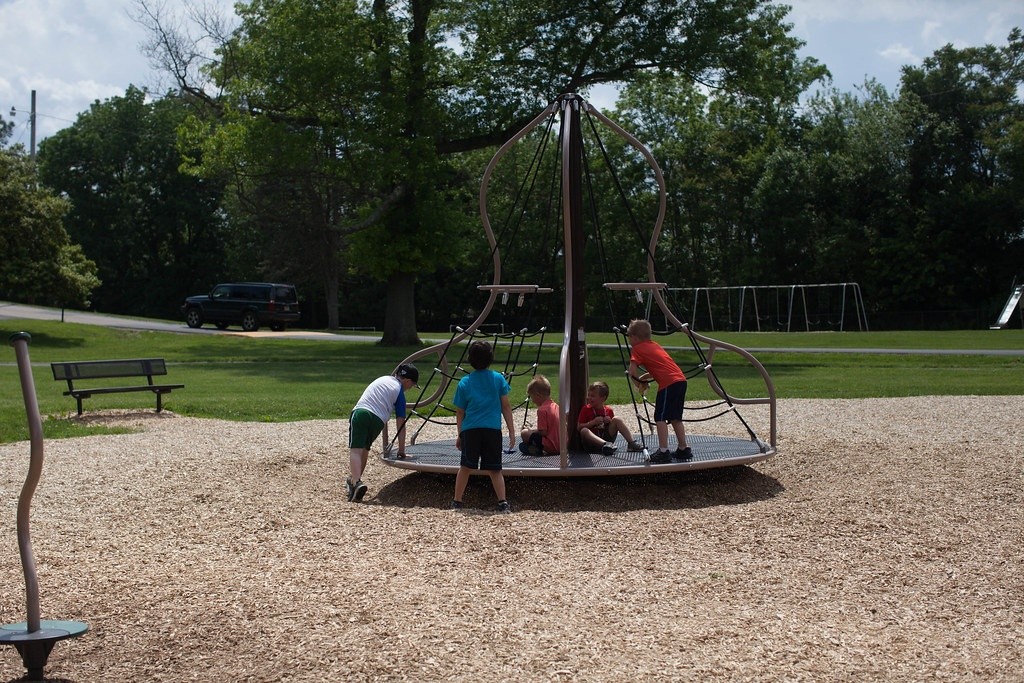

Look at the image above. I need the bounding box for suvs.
[181,282,303,332]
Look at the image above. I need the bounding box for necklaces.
[592,403,606,429]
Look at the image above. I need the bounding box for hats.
[396,364,421,390]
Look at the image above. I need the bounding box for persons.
[347,362,418,502]
[576,381,648,456]
[627,320,693,462]
[449,340,515,513]
[518,373,560,457]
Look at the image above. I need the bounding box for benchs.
[450,325,505,335]
[339,327,375,333]
[50,359,185,415]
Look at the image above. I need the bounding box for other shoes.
[347,478,361,497]
[627,439,648,452]
[650,448,673,462]
[494,500,511,513]
[450,497,463,509]
[349,481,367,502]
[602,441,618,456]
[672,445,693,458]
